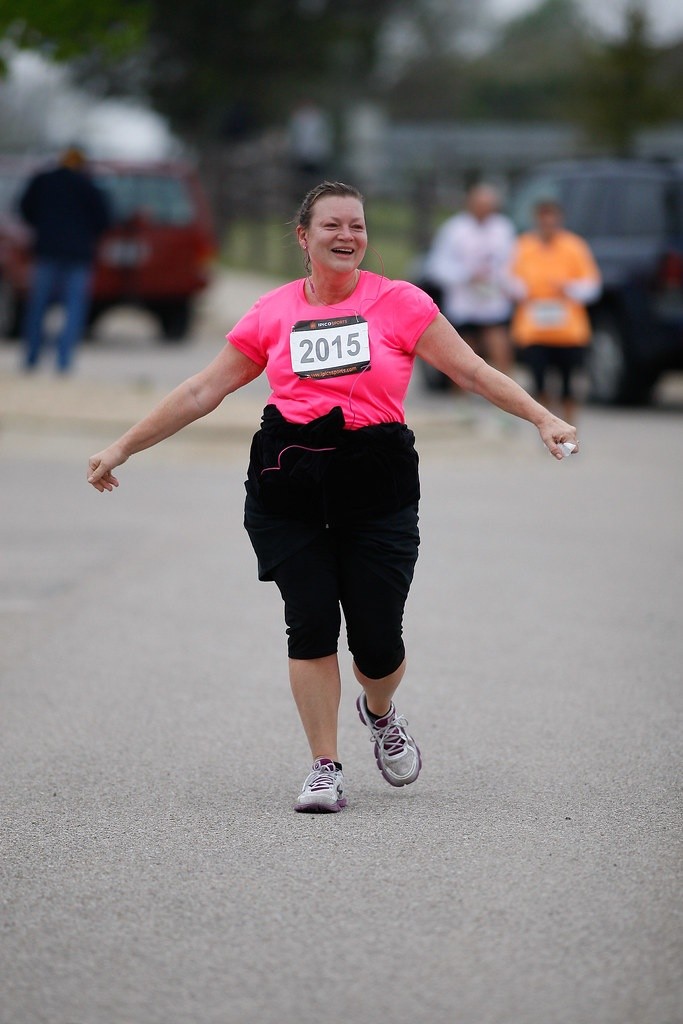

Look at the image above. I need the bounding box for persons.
[496,199,601,422]
[15,145,114,371]
[429,177,517,400]
[87,182,580,813]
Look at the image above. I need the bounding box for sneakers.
[356,689,422,787]
[295,758,346,812]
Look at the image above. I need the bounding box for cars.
[402,156,683,408]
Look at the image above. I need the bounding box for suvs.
[1,141,218,342]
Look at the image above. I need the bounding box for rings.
[577,441,579,443]
